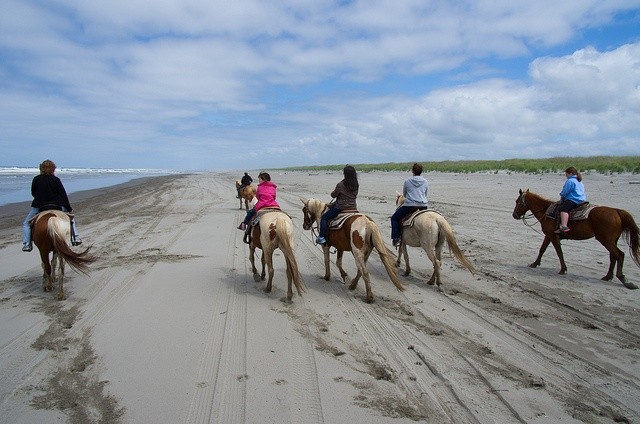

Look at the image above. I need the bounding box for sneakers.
[392,237,401,246]
[555,227,570,234]
[71,236,82,243]
[316,236,326,244]
[22,244,33,251]
[237,223,246,230]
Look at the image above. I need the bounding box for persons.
[237,171,253,197]
[553,167,586,233]
[389,162,428,246]
[315,164,358,243]
[21,160,81,251]
[239,173,281,230]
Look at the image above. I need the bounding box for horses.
[236,180,256,212]
[512,188,640,289]
[31,210,95,301]
[249,212,305,304]
[396,191,475,291]
[300,198,404,303]
